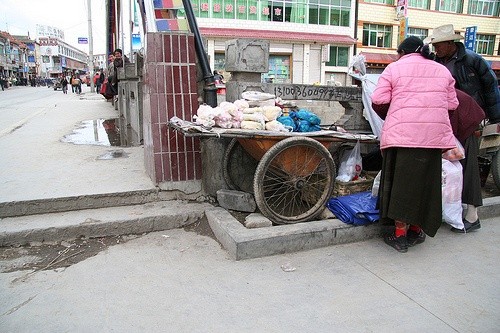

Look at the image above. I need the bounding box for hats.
[423,24,464,45]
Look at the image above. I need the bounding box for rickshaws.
[166,131,380,226]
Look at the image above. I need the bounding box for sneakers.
[384,231,409,253]
[407,229,426,247]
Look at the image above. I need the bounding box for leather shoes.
[452,218,480,232]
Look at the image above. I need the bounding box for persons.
[448,88,486,234]
[370,36,460,253]
[99,49,130,100]
[0,69,103,96]
[422,24,500,174]
